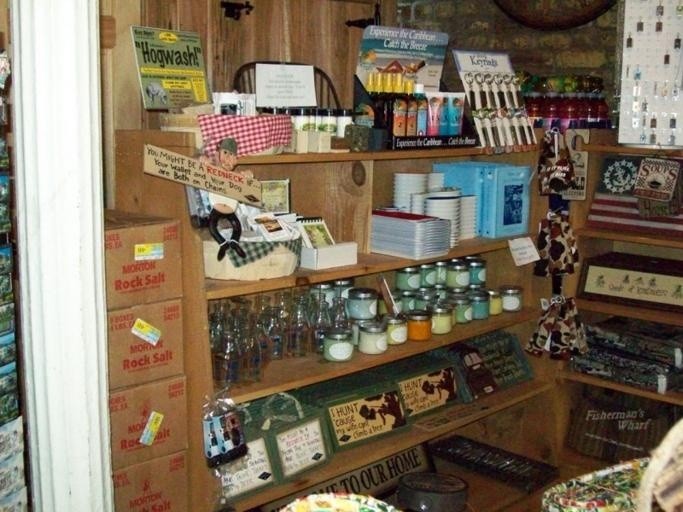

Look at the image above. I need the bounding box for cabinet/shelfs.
[111,128,559,512]
[559,127,683,479]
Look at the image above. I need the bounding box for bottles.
[446,264,470,288]
[320,108,337,131]
[485,288,503,315]
[404,79,417,137]
[355,110,369,126]
[275,293,282,307]
[334,280,358,300]
[395,267,422,292]
[309,108,321,130]
[450,296,472,325]
[466,290,490,321]
[358,319,388,355]
[380,296,403,317]
[268,318,283,360]
[448,258,466,264]
[383,313,409,346]
[400,291,420,312]
[468,259,487,285]
[314,302,332,354]
[426,303,453,335]
[257,322,268,360]
[293,288,312,297]
[435,262,449,286]
[322,327,354,362]
[419,264,437,288]
[597,94,610,127]
[388,72,405,137]
[263,108,270,115]
[288,297,308,357]
[283,293,291,313]
[543,91,560,127]
[310,284,337,305]
[347,288,378,320]
[258,294,271,326]
[415,84,429,137]
[283,106,290,114]
[466,255,481,262]
[366,74,392,127]
[407,309,432,342]
[209,334,219,380]
[333,297,347,331]
[577,92,590,119]
[430,285,447,305]
[272,307,281,317]
[337,108,352,137]
[351,319,374,348]
[414,288,432,310]
[217,332,238,385]
[243,327,263,382]
[592,96,603,119]
[316,108,328,131]
[294,109,310,130]
[560,92,576,129]
[499,285,524,313]
[525,91,541,128]
[207,296,257,334]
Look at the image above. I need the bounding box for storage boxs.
[110,376,189,470]
[111,448,190,512]
[108,300,191,391]
[105,211,184,311]
